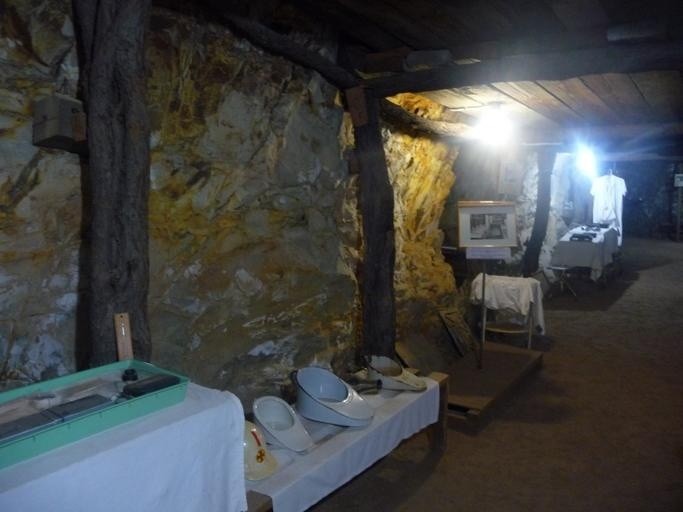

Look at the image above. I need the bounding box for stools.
[545,265,577,300]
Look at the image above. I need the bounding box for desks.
[553,224,620,286]
[245,366,448,512]
[0,381,244,512]
